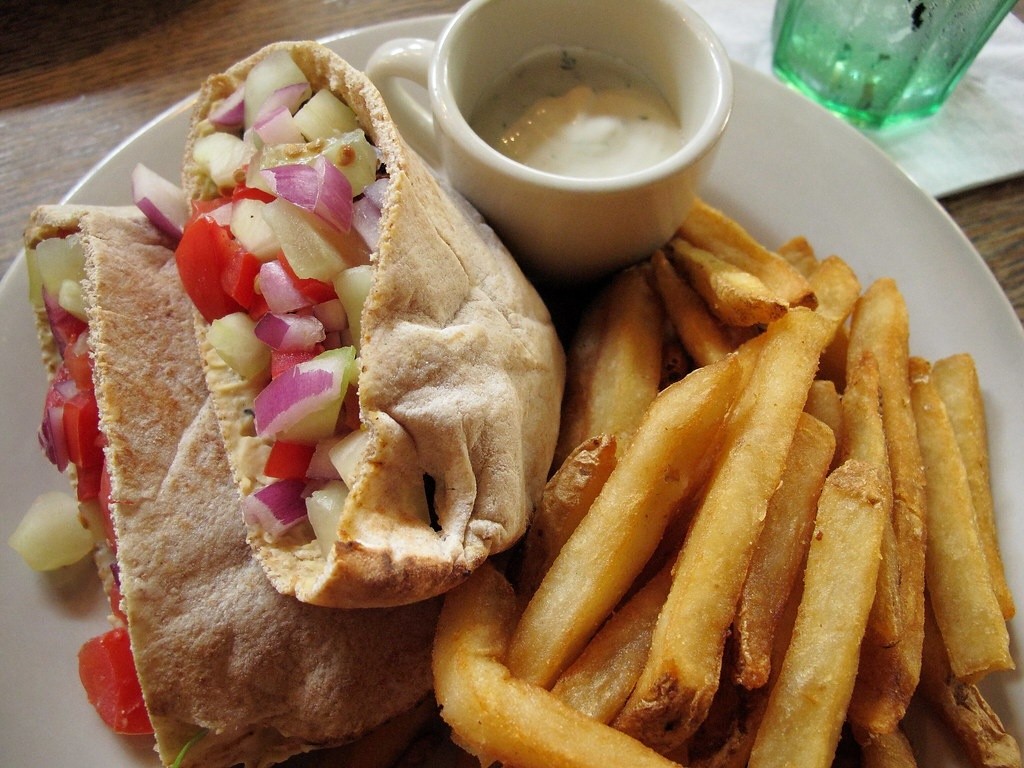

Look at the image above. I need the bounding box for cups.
[771,1,1019,131]
[362,0,734,284]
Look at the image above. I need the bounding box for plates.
[0,12,1024,768]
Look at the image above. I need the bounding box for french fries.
[432,198,1024,767]
